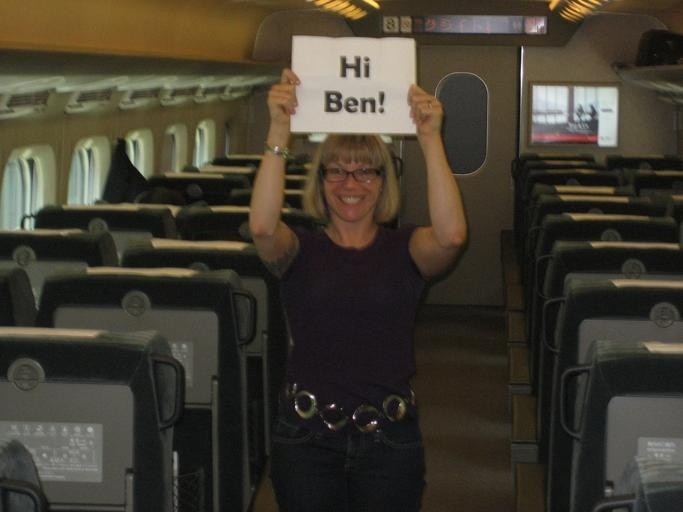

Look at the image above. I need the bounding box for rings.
[427,101,433,109]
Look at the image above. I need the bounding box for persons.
[249,68,469,512]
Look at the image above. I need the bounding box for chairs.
[2,149,405,511]
[510,151,683,512]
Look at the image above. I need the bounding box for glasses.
[321,167,384,182]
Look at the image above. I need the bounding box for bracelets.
[263,141,294,160]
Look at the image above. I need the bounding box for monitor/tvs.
[528,81,619,150]
